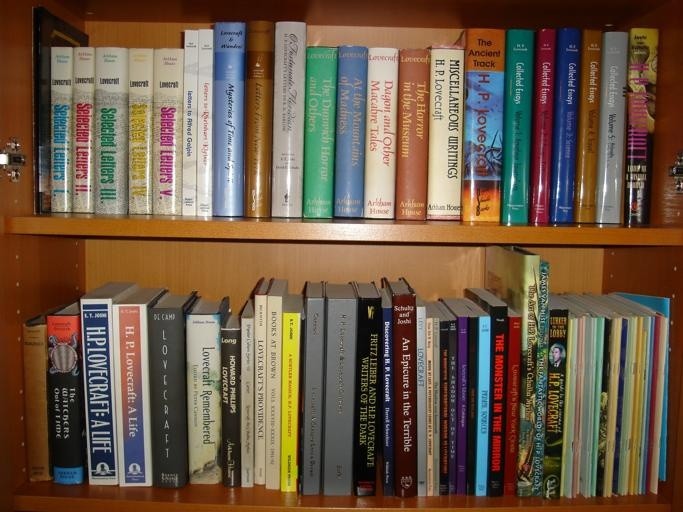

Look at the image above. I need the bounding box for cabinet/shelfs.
[0,0,683,512]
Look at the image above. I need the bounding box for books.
[24,245,671,499]
[32,8,659,224]
